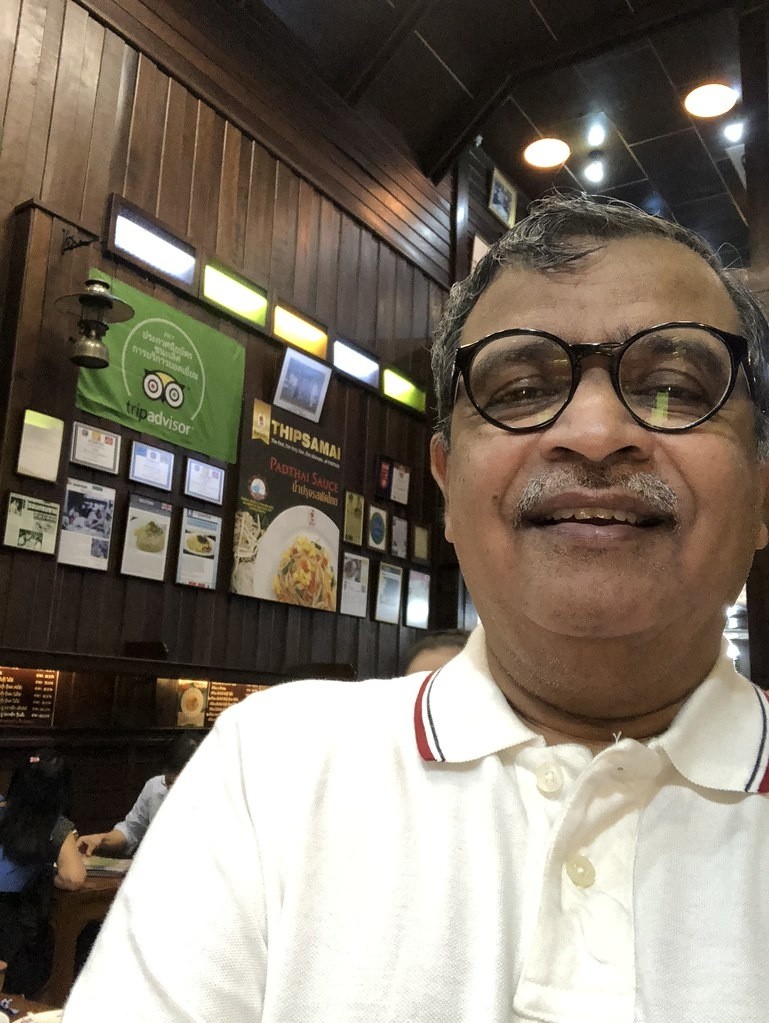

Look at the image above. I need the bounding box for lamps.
[52,236,135,369]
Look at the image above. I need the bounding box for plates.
[180,687,204,718]
[11,1009,63,1023]
[183,505,340,613]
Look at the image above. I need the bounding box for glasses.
[448,320,769,434]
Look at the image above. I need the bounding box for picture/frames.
[272,347,335,425]
[372,560,403,625]
[485,166,518,230]
[339,551,370,618]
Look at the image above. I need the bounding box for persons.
[0,764,87,1001]
[397,627,467,679]
[73,737,198,982]
[62,194,769,1023]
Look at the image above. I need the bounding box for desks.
[41,869,123,1005]
